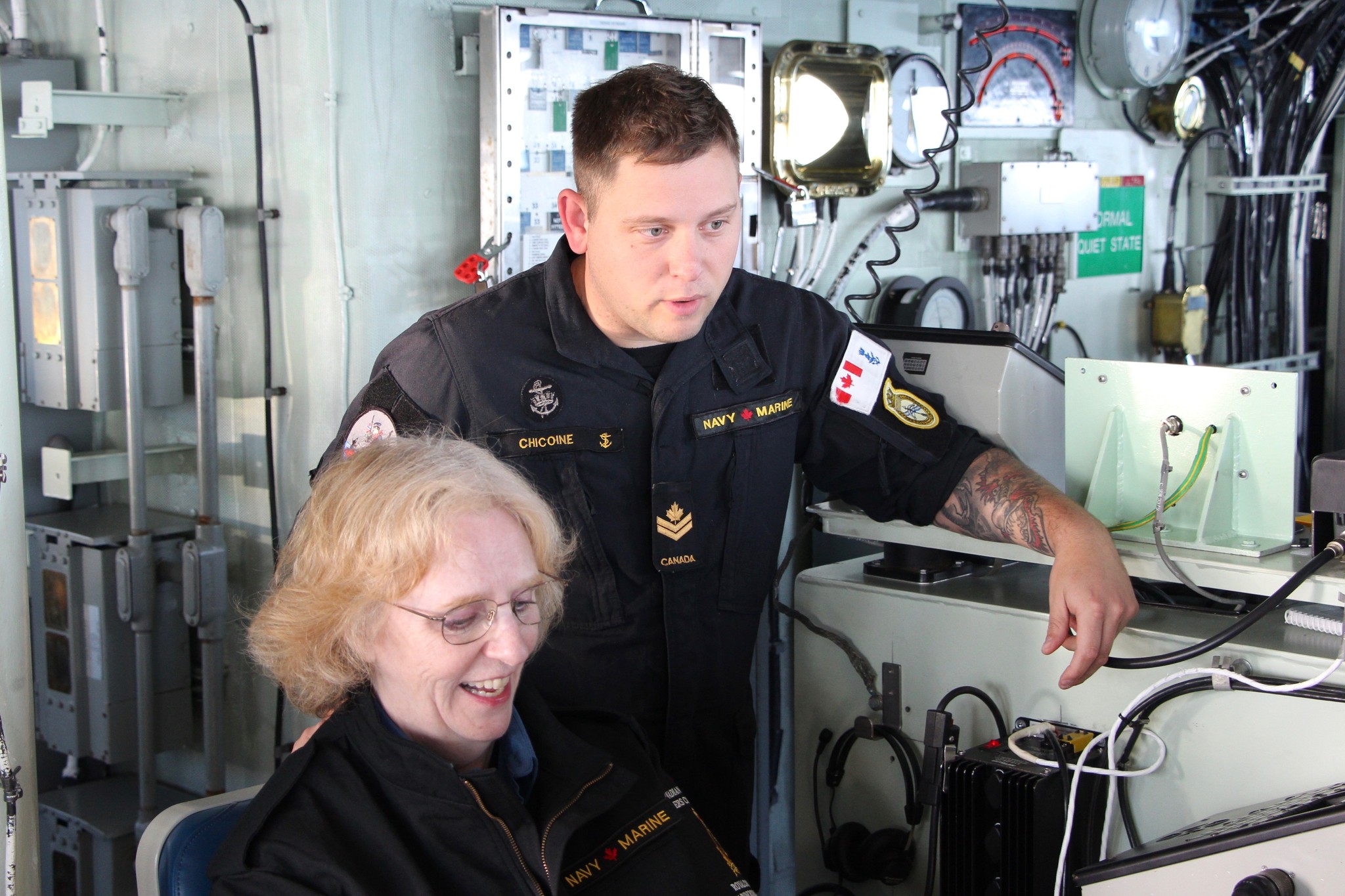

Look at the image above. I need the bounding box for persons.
[208,432,754,896]
[311,64,1141,895]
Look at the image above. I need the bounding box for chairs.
[133,783,267,896]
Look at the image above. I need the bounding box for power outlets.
[1014,717,1109,751]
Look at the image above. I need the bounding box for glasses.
[382,571,568,644]
